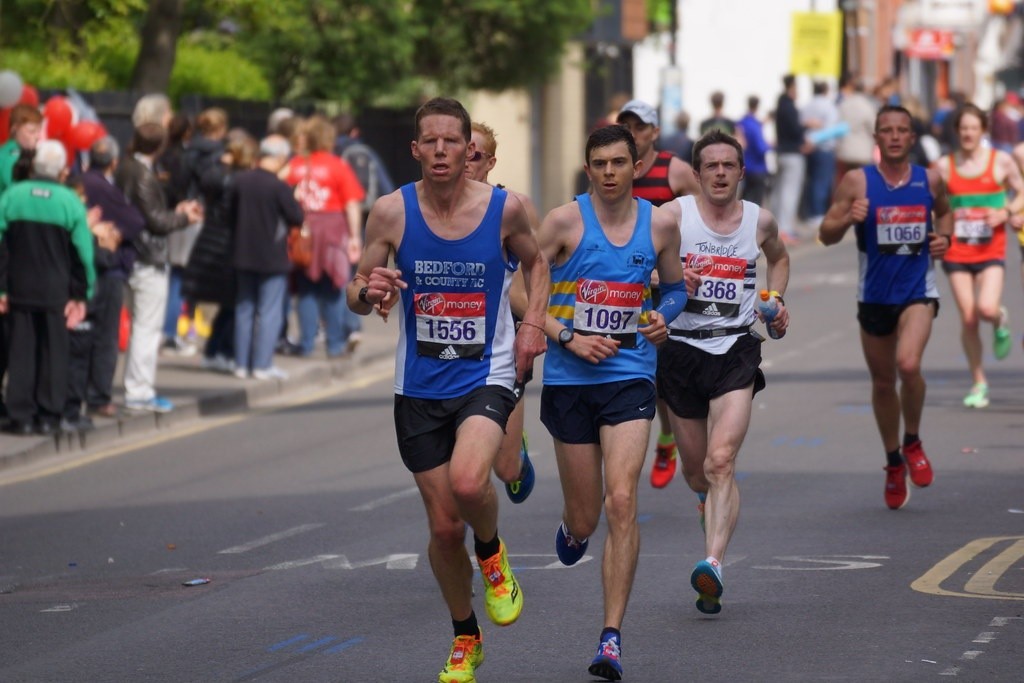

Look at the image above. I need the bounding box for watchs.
[559,327,574,348]
[359,287,368,304]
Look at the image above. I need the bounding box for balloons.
[0,66,104,172]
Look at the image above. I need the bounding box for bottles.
[757,289,787,339]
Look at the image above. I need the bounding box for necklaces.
[877,162,912,192]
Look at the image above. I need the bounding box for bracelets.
[770,290,785,306]
[515,321,544,332]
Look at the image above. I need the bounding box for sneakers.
[589,635,623,682]
[691,559,724,615]
[902,440,933,488]
[882,456,910,511]
[650,442,679,489]
[963,384,990,408]
[994,314,1013,361]
[503,432,535,503]
[438,627,485,683]
[695,492,707,534]
[474,531,523,625]
[555,515,589,566]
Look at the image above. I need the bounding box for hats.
[616,99,657,129]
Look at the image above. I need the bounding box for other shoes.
[125,394,174,413]
[236,363,283,381]
[276,339,303,356]
[204,352,238,373]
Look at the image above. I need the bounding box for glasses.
[469,151,494,162]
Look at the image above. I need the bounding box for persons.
[660,128,791,617]
[462,72,1024,510]
[0,94,399,436]
[506,126,689,681]
[347,101,552,683]
